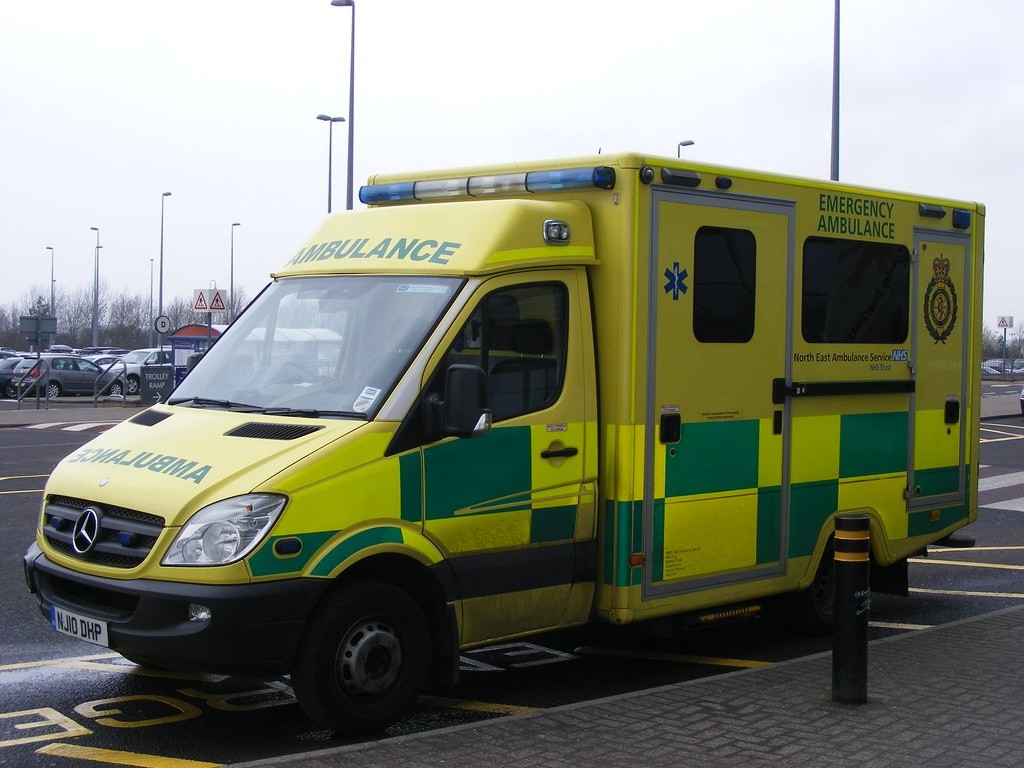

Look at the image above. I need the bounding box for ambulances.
[22,151,986,737]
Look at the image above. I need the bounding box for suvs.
[108,348,172,395]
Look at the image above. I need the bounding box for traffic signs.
[139,365,174,406]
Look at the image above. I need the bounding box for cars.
[981,359,1024,376]
[0,346,24,386]
[44,345,131,370]
[11,354,129,398]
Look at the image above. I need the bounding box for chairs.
[483,318,560,423]
[369,314,431,393]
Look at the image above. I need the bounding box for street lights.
[207,280,218,347]
[91,246,103,347]
[149,258,154,348]
[155,192,171,348]
[53,280,56,316]
[332,1,355,209]
[678,140,695,158]
[90,227,99,347]
[230,223,240,324]
[46,247,54,316]
